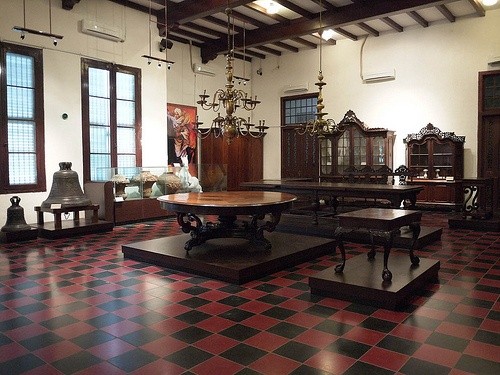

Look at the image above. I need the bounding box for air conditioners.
[363,68,395,82]
[193,63,218,77]
[284,82,309,93]
[80,18,126,43]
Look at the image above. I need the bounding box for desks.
[157,191,297,257]
[239,181,426,226]
[334,207,422,281]
[34,204,100,229]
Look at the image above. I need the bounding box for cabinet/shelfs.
[320,110,396,184]
[198,128,267,192]
[402,123,465,212]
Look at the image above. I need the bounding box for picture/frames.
[167,103,198,178]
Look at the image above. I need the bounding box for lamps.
[192,0,269,144]
[294,0,351,140]
